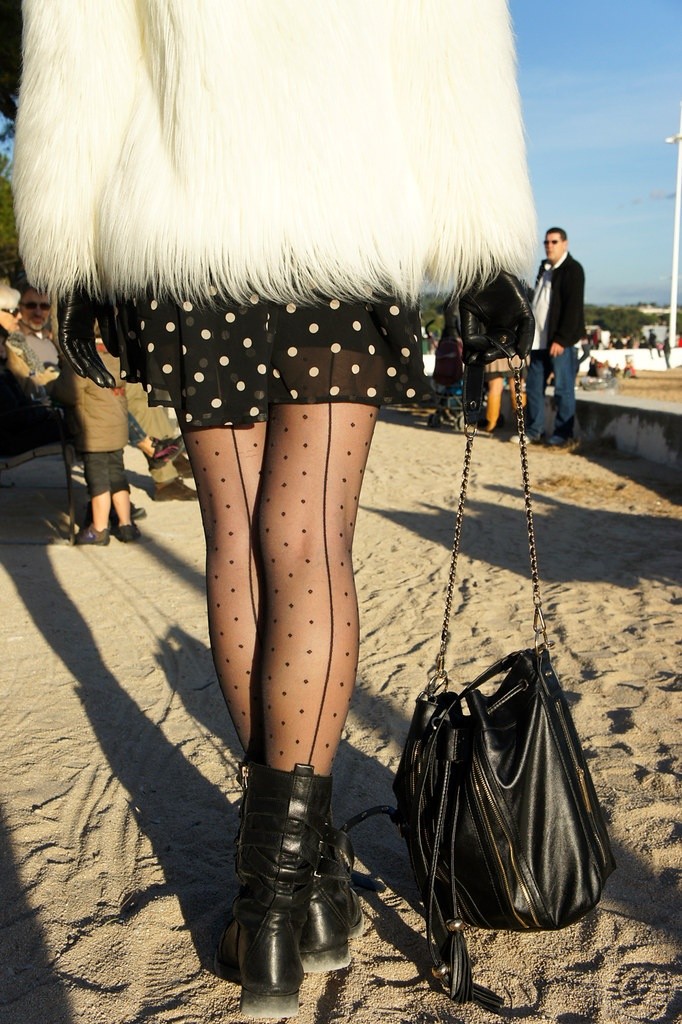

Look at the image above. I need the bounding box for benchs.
[0,404,88,545]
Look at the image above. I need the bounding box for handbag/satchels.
[341,348,614,1015]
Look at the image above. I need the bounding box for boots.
[296,806,365,974]
[214,761,334,1018]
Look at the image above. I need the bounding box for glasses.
[2,308,20,317]
[544,240,558,245]
[21,301,52,311]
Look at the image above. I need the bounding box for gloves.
[457,266,536,372]
[56,283,120,390]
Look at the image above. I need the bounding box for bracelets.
[28,370,35,376]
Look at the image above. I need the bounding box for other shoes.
[177,460,194,478]
[129,501,146,518]
[112,522,140,541]
[548,435,566,444]
[510,431,544,445]
[148,435,184,473]
[153,474,199,502]
[73,523,110,546]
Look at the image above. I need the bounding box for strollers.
[423,320,465,431]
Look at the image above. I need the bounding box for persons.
[585,328,682,377]
[511,228,585,445]
[0,279,198,544]
[15,1,533,1016]
[432,309,529,434]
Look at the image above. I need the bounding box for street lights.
[666,134,681,348]
